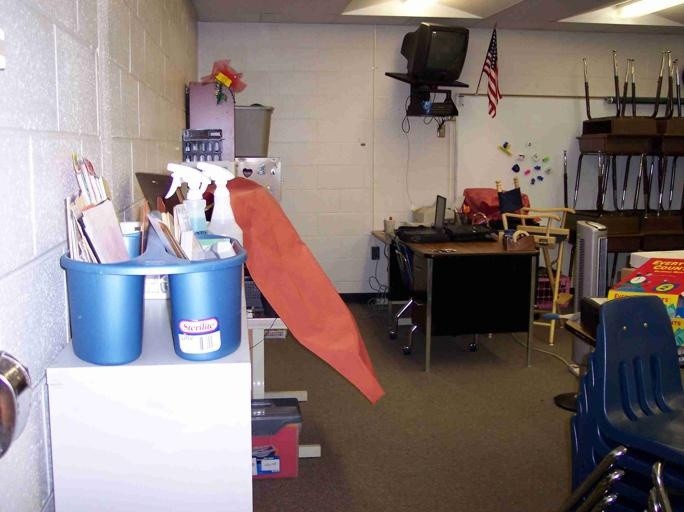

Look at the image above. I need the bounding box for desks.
[563,50,683,292]
[373,231,539,377]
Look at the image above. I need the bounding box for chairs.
[495,177,577,347]
[555,294,684,511]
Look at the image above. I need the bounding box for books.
[65,154,130,264]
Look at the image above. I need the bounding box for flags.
[483,29,502,118]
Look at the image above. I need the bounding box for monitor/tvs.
[401,22,469,84]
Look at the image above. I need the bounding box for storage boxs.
[233,103,274,157]
[251,399,301,480]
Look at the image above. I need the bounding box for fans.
[572,216,610,365]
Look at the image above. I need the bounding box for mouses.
[410,235,424,241]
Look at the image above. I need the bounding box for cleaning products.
[162,161,211,237]
[196,162,243,250]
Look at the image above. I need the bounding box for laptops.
[404,195,447,235]
